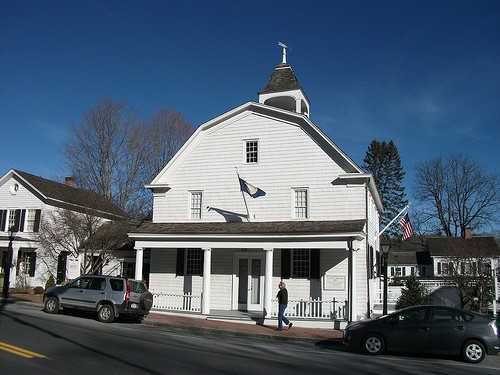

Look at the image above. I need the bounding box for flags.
[398,211,415,242]
[239,177,267,199]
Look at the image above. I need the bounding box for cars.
[343,304,500,364]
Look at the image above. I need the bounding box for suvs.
[42,274,154,323]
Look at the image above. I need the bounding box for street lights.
[2,225,19,298]
[379,243,392,315]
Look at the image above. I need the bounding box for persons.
[57,270,63,284]
[274,282,293,331]
[44,274,56,291]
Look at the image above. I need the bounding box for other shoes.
[288,323,292,329]
[274,328,283,331]
